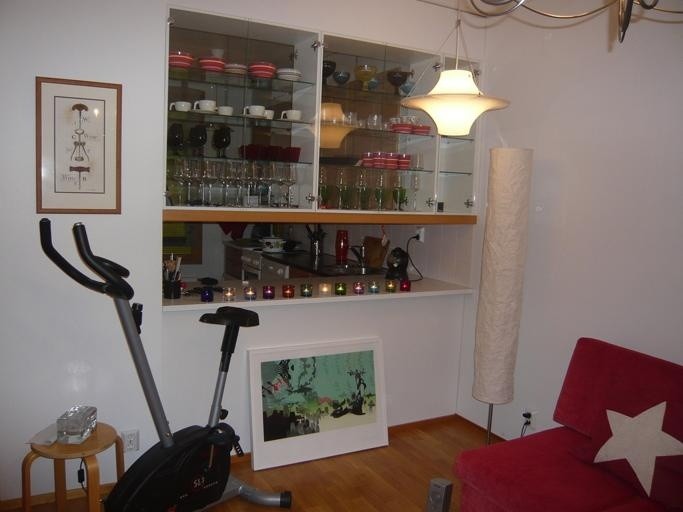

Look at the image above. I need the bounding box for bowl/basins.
[272,65,302,81]
[393,122,430,137]
[246,60,275,78]
[169,50,195,69]
[196,56,226,71]
[221,62,247,74]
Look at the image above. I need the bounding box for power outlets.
[416,226,425,243]
[521,407,540,428]
[122,430,139,452]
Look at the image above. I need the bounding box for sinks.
[321,264,385,276]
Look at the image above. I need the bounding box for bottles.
[335,229,349,263]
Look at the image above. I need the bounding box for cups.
[310,239,322,255]
[242,104,275,120]
[169,99,191,112]
[199,278,410,302]
[280,108,301,120]
[163,279,180,299]
[193,99,217,111]
[239,141,303,163]
[360,151,423,169]
[320,107,389,130]
[215,105,232,116]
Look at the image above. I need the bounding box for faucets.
[347,245,367,266]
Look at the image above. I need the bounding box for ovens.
[241,264,261,280]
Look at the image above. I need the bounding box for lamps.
[471,147,534,445]
[222,280,411,301]
[400,0,509,137]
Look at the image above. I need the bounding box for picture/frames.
[247,336,389,471]
[35,75,121,213]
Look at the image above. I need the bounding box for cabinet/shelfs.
[289,267,322,280]
[163,4,483,224]
[223,245,242,277]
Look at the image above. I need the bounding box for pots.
[258,236,286,253]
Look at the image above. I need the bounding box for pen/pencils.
[164,252,183,282]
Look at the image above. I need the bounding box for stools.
[22,422,124,512]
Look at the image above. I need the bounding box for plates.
[238,113,264,120]
[192,107,215,114]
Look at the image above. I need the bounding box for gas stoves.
[239,246,293,264]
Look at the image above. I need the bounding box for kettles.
[385,247,409,280]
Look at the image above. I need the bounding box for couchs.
[452,337,683,512]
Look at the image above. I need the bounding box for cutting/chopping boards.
[361,235,391,271]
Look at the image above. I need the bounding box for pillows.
[581,382,683,512]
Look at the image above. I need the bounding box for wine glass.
[334,166,349,209]
[166,158,297,207]
[318,166,327,210]
[407,172,423,212]
[169,120,233,160]
[372,170,387,211]
[389,173,405,213]
[322,59,413,97]
[353,168,369,211]
